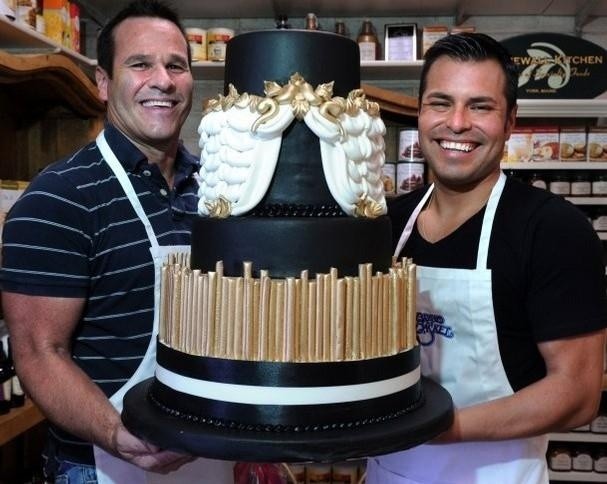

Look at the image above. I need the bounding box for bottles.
[274,7,381,62]
[1,335,26,417]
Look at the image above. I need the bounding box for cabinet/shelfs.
[2,396,48,446]
[0,11,105,218]
[503,98,607,240]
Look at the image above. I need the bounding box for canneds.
[506,171,607,196]
[550,416,607,473]
[382,128,425,195]
[583,208,607,241]
[183,28,234,60]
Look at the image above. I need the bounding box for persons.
[359,32,607,484]
[0,0,238,484]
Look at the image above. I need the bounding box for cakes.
[147,28,425,432]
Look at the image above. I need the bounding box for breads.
[560,144,585,158]
[541,143,559,159]
[588,142,607,161]
